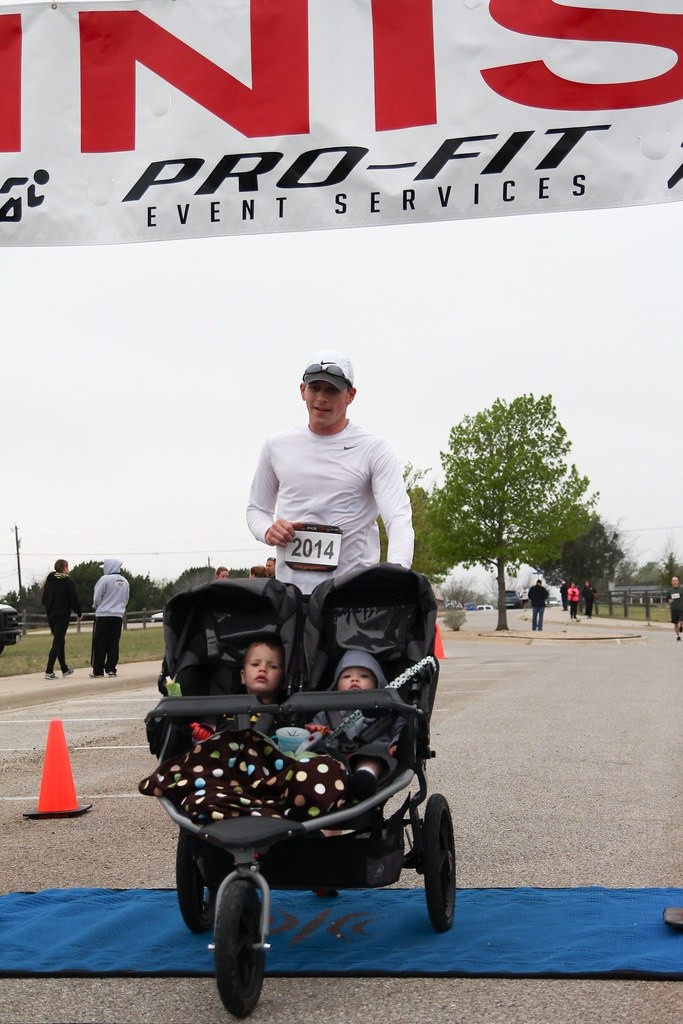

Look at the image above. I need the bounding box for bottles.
[164,676,181,697]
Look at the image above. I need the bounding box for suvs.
[505,590,523,609]
[0,604,21,655]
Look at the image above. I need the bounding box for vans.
[477,605,493,610]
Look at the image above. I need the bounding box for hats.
[326,650,388,691]
[301,350,354,392]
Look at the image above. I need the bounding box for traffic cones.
[434,624,449,660]
[23,720,94,821]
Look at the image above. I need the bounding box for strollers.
[143,561,457,1020]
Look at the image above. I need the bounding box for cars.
[464,602,477,610]
[544,596,560,607]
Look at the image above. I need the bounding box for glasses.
[267,557,275,561]
[302,363,353,389]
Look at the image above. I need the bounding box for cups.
[270,726,311,753]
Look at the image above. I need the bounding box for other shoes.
[89,672,104,678]
[108,671,117,677]
[44,673,59,680]
[63,669,74,676]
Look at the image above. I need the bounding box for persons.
[211,629,287,737]
[266,557,276,578]
[216,566,229,580]
[581,580,597,619]
[520,587,529,611]
[306,649,407,802]
[528,580,549,631]
[247,351,415,897]
[567,582,580,619]
[41,559,82,679]
[248,565,266,578]
[88,559,130,677]
[664,576,683,640]
[560,580,569,610]
